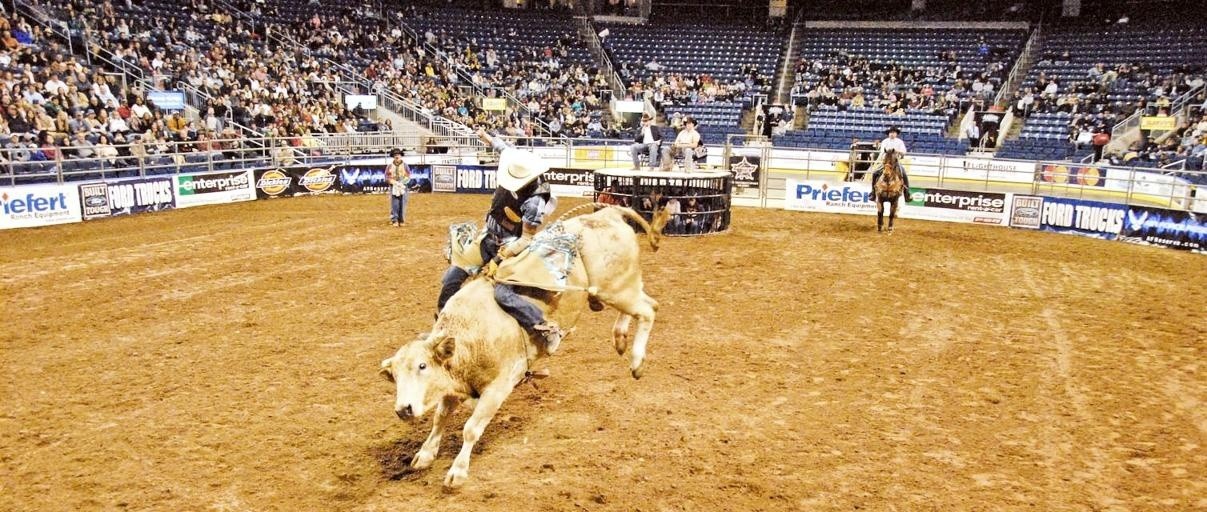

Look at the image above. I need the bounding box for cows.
[377,204,670,489]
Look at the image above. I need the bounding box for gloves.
[481,256,502,277]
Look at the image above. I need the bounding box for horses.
[873,147,905,233]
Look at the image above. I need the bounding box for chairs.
[0,0,1207,190]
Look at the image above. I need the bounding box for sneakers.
[533,319,564,355]
[905,196,912,202]
[869,194,875,200]
[632,167,639,170]
[686,167,691,172]
[393,222,406,227]
[660,168,672,171]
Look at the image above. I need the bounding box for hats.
[684,117,697,126]
[498,149,550,192]
[391,149,404,154]
[641,114,653,121]
[886,126,900,135]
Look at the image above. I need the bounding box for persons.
[619,38,1207,170]
[434,126,563,356]
[866,123,914,203]
[0,0,619,184]
[386,148,409,228]
